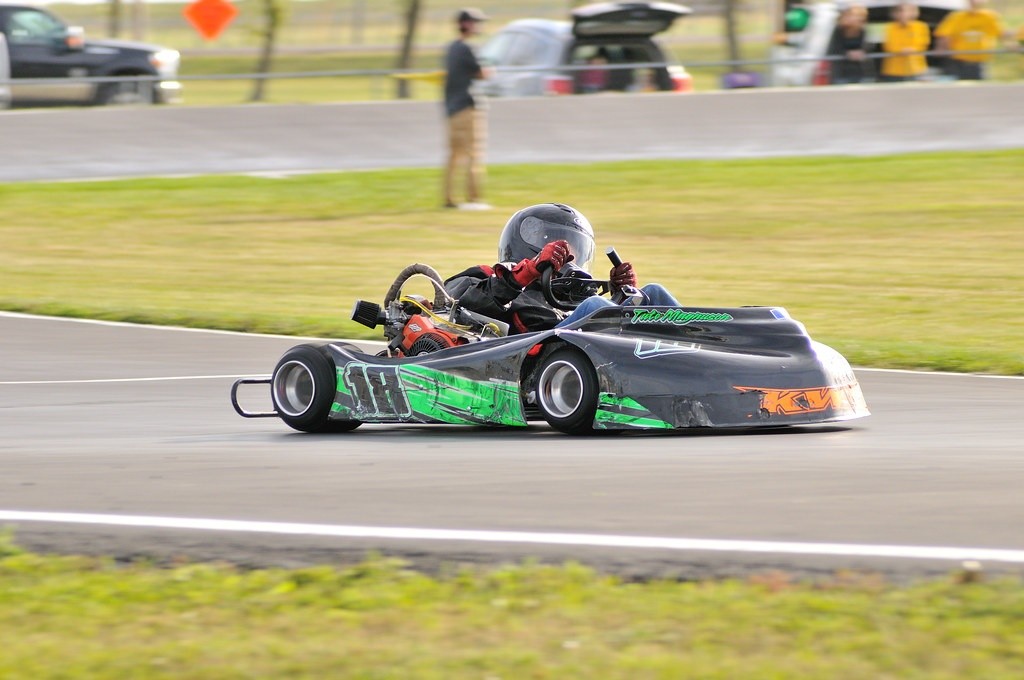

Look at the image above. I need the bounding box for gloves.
[609,262,636,296]
[527,239,570,272]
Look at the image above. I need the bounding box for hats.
[459,9,490,22]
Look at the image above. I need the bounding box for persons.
[932,0,1019,80]
[579,47,611,94]
[442,6,496,211]
[831,6,876,84]
[443,203,682,333]
[880,5,931,82]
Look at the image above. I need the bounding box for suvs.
[468,0,692,94]
[0,4,179,105]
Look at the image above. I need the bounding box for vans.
[775,0,983,86]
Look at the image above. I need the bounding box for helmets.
[497,203,594,274]
